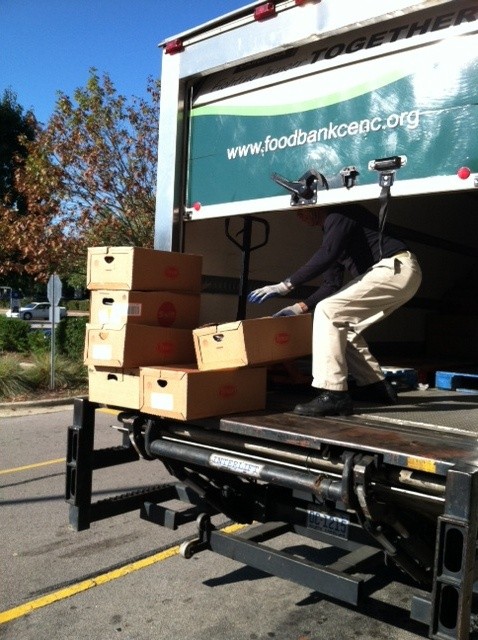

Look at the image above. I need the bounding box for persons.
[246,207,422,416]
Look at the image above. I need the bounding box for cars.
[7,302,67,320]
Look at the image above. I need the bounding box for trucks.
[68,0,477,639]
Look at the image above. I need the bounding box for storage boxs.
[139,366,268,423]
[90,289,201,328]
[85,245,203,294]
[192,314,313,371]
[85,323,193,368]
[88,367,142,410]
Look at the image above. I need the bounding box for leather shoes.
[351,387,398,404]
[293,391,352,417]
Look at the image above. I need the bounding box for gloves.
[247,280,292,304]
[271,302,304,316]
